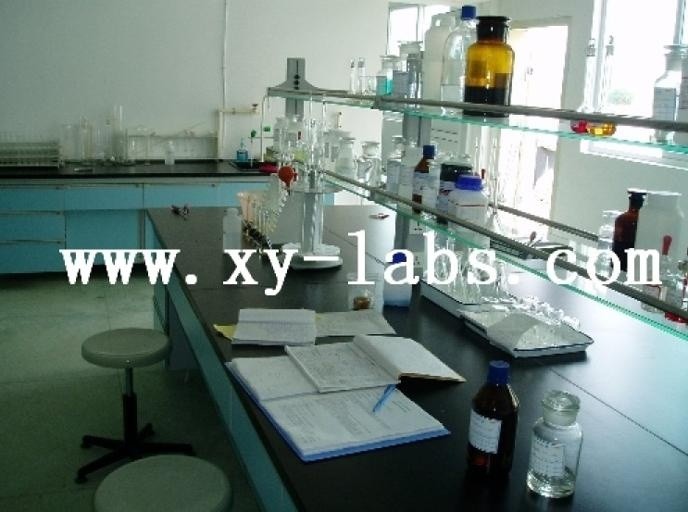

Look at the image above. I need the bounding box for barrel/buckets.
[421,14,457,109]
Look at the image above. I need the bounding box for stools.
[90,451,232,511]
[74,326,197,484]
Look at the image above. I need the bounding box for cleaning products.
[237,136,248,161]
[164,140,175,166]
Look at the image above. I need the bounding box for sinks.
[229,160,266,171]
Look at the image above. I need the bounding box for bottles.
[528,388,586,501]
[222,206,242,254]
[356,138,384,187]
[386,134,490,242]
[51,101,180,169]
[568,36,620,138]
[427,234,581,350]
[382,251,415,305]
[237,109,356,237]
[345,4,516,124]
[591,188,687,325]
[464,358,521,478]
[652,42,688,150]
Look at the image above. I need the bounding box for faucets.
[259,95,270,162]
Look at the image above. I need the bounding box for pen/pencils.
[372,384,397,412]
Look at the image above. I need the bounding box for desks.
[139,202,686,512]
[301,202,688,458]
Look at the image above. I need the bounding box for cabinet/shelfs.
[264,83,687,325]
[0,159,279,273]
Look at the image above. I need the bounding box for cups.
[343,274,379,313]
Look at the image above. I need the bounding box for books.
[283,332,468,394]
[222,353,453,465]
[231,308,317,347]
